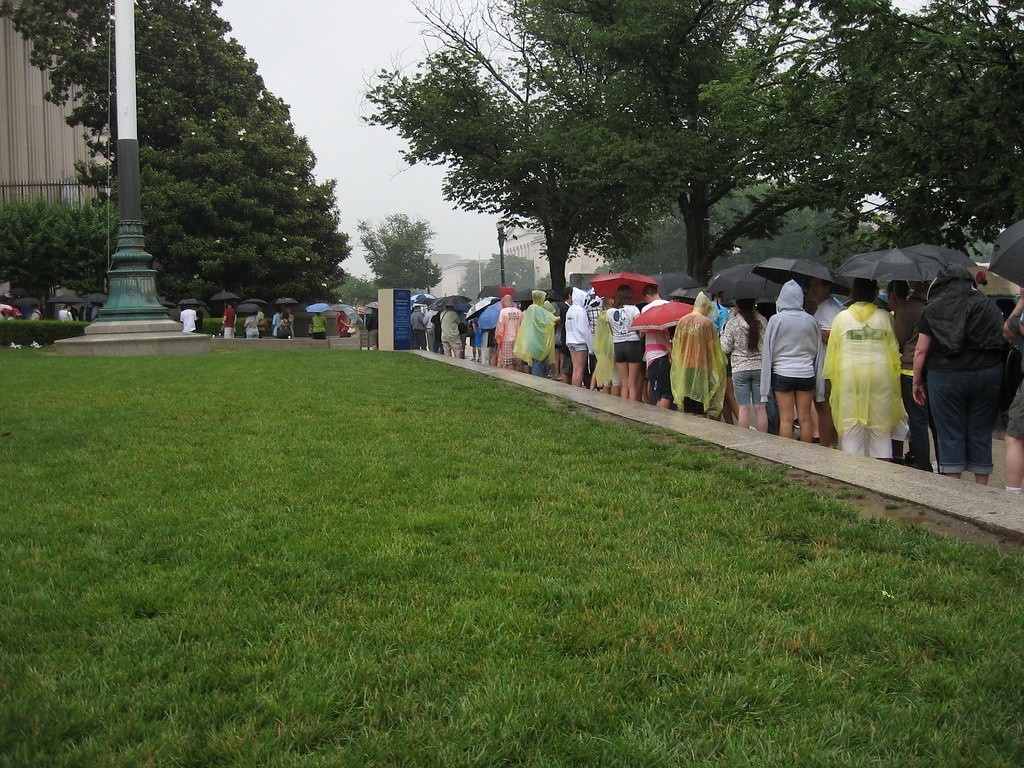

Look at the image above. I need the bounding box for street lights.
[424,253,431,294]
[462,288,466,296]
[495,217,507,287]
[511,281,517,289]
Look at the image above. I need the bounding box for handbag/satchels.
[277,319,291,339]
[309,324,314,334]
[555,325,562,345]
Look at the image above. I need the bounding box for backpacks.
[32,310,45,320]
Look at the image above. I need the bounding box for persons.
[272,306,295,339]
[80,302,103,322]
[366,307,378,351]
[410,278,1024,493]
[311,312,327,340]
[58,303,79,321]
[243,310,265,339]
[180,305,204,333]
[335,311,356,338]
[44,303,54,320]
[0,304,41,320]
[219,301,237,338]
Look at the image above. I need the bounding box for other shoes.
[471,358,476,362]
[477,358,483,362]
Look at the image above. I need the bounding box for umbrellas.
[365,301,377,309]
[161,301,177,309]
[270,297,298,309]
[179,299,206,305]
[86,293,109,302]
[409,219,1024,310]
[46,295,85,303]
[304,303,351,313]
[210,289,241,309]
[346,308,363,322]
[11,298,42,303]
[237,298,268,313]
